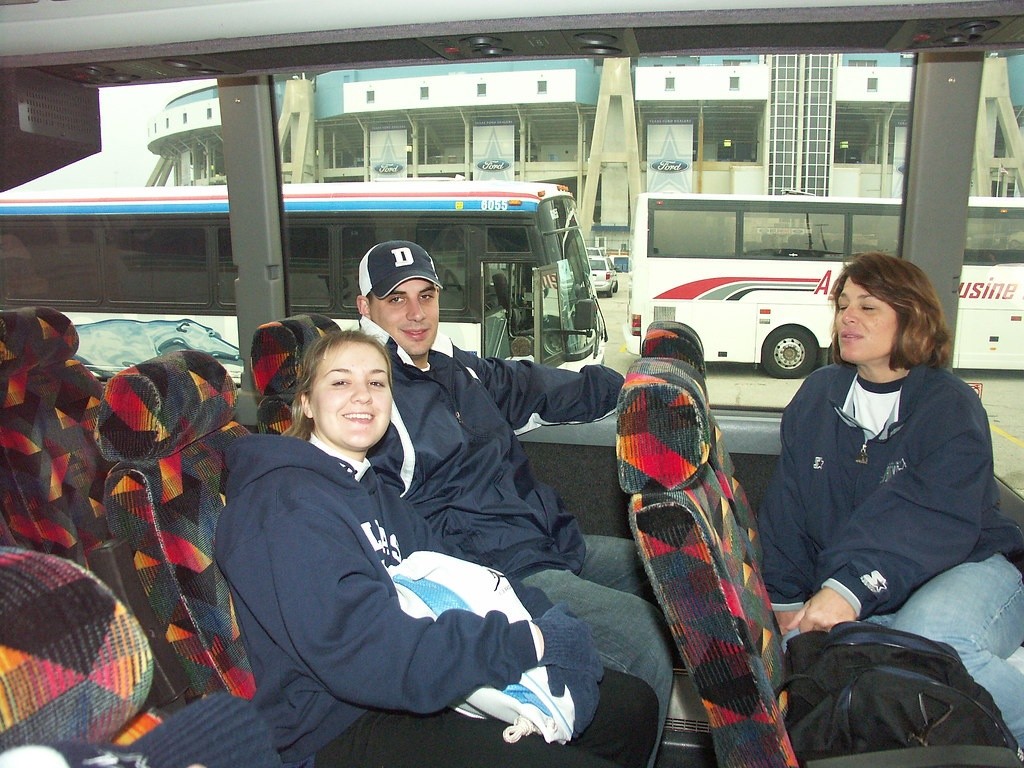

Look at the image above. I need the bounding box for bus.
[622,192,1024,379]
[0,191,608,389]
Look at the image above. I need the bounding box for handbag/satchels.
[774,621,1022,763]
[387,550,574,745]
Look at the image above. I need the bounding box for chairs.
[94,348,258,714]
[250,313,341,435]
[641,322,764,579]
[745,234,844,255]
[615,358,1024,768]
[0,306,119,573]
[0,546,285,768]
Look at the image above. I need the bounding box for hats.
[357,240,443,300]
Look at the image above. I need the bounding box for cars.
[608,255,632,274]
[588,255,620,298]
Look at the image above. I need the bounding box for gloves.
[530,603,606,681]
[545,666,598,735]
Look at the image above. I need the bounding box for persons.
[214,331,659,768]
[758,250,1024,755]
[354,239,677,768]
